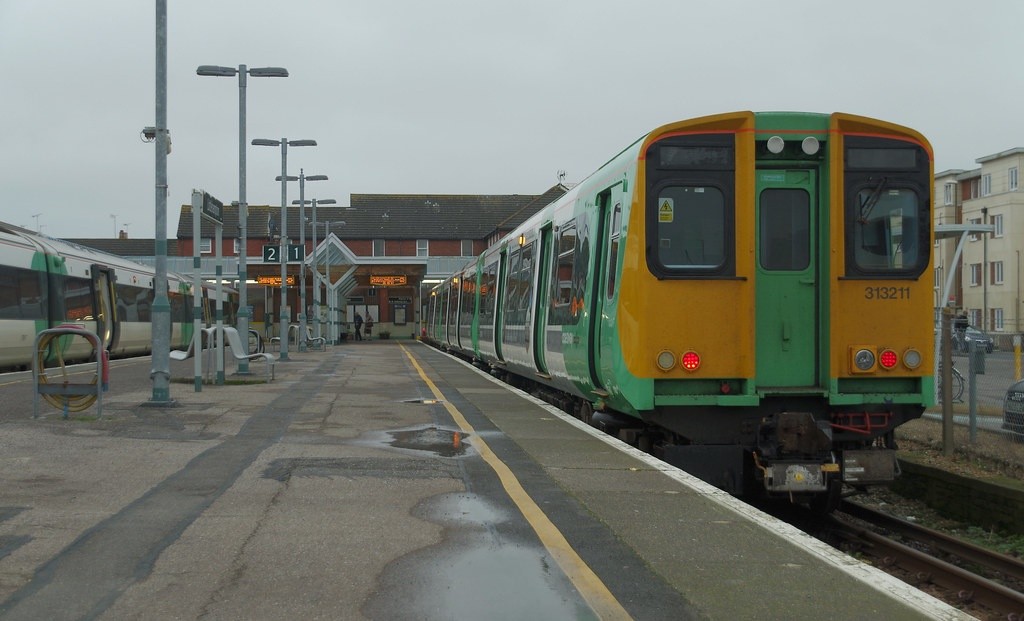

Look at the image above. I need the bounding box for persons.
[953,310,970,353]
[354,312,363,341]
[363,310,374,341]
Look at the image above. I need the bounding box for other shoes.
[363,339,366,340]
[368,339,372,341]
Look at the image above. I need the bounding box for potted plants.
[379,331,390,340]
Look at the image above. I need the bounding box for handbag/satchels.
[365,316,373,327]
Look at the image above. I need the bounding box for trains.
[425,111,929,486]
[0,223,239,365]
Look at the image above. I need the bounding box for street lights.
[292,198,336,354]
[196,65,287,376]
[318,221,345,347]
[251,139,317,359]
[275,169,328,353]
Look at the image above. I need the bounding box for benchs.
[170,325,325,383]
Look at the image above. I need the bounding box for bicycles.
[937,361,965,404]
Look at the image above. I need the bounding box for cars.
[935,319,993,353]
[1001,381,1024,434]
